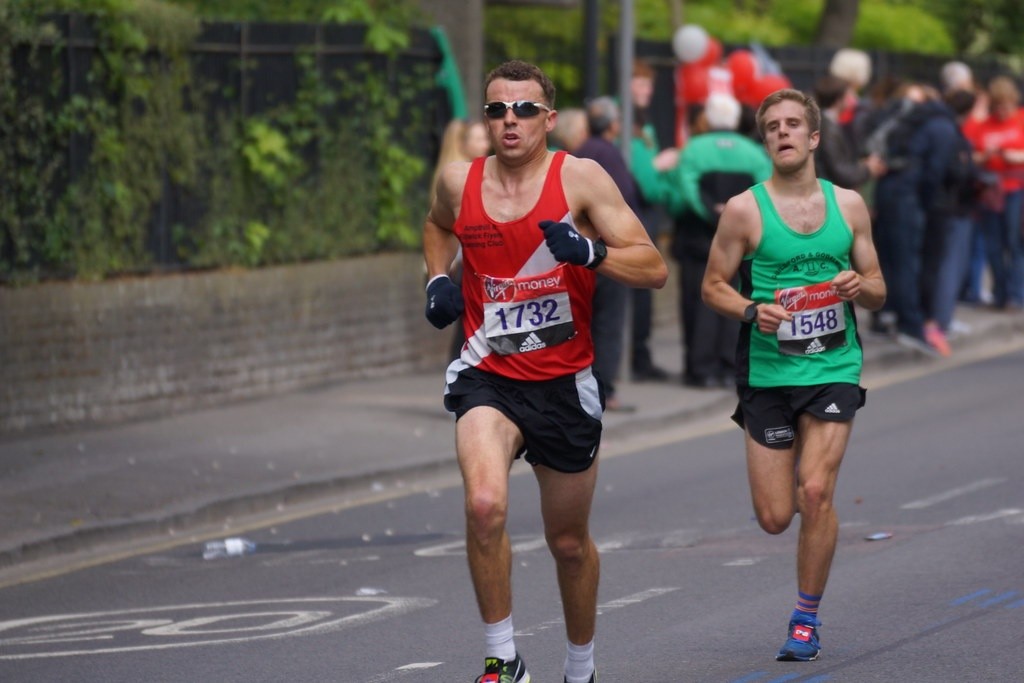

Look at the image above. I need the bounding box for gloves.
[538,220,594,266]
[426,274,465,329]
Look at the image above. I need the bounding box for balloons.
[672,24,791,106]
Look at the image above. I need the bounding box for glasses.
[483,101,549,119]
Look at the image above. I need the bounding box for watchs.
[744,302,758,323]
[585,240,608,270]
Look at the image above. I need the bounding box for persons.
[421,60,669,683]
[700,88,887,662]
[430,49,1023,413]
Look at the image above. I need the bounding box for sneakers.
[475,651,530,683]
[775,614,823,660]
[564,668,597,683]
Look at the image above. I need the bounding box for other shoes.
[897,329,939,360]
[636,366,668,380]
[605,398,639,411]
